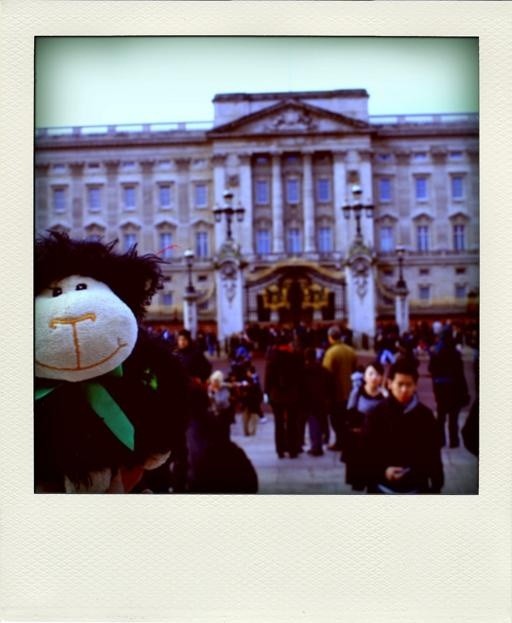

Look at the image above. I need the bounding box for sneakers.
[441,438,462,450]
[276,443,342,460]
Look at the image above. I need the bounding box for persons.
[143,320,479,458]
[365,362,443,494]
[341,364,393,490]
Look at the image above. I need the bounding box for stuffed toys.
[35,226,185,493]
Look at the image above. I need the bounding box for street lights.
[183,248,194,293]
[213,190,244,241]
[395,243,405,288]
[341,185,374,235]
